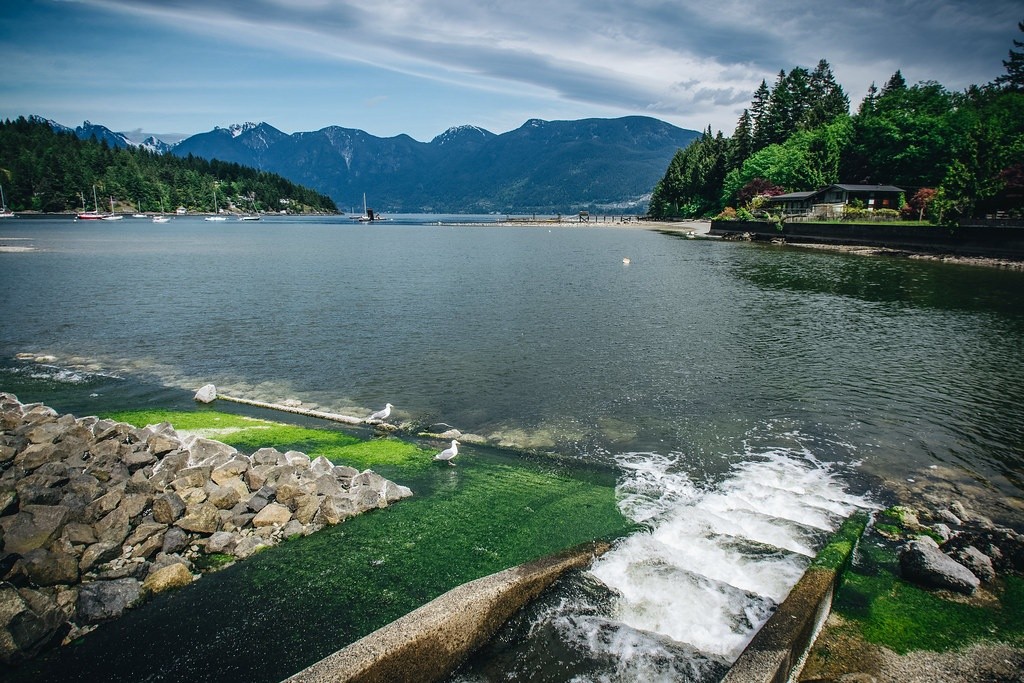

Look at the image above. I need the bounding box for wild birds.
[369,403,393,420]
[433,439,462,463]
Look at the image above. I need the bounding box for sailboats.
[77,185,124,220]
[151,197,175,222]
[348,193,387,221]
[204,192,226,221]
[0,185,14,218]
[238,191,261,220]
[131,201,147,218]
